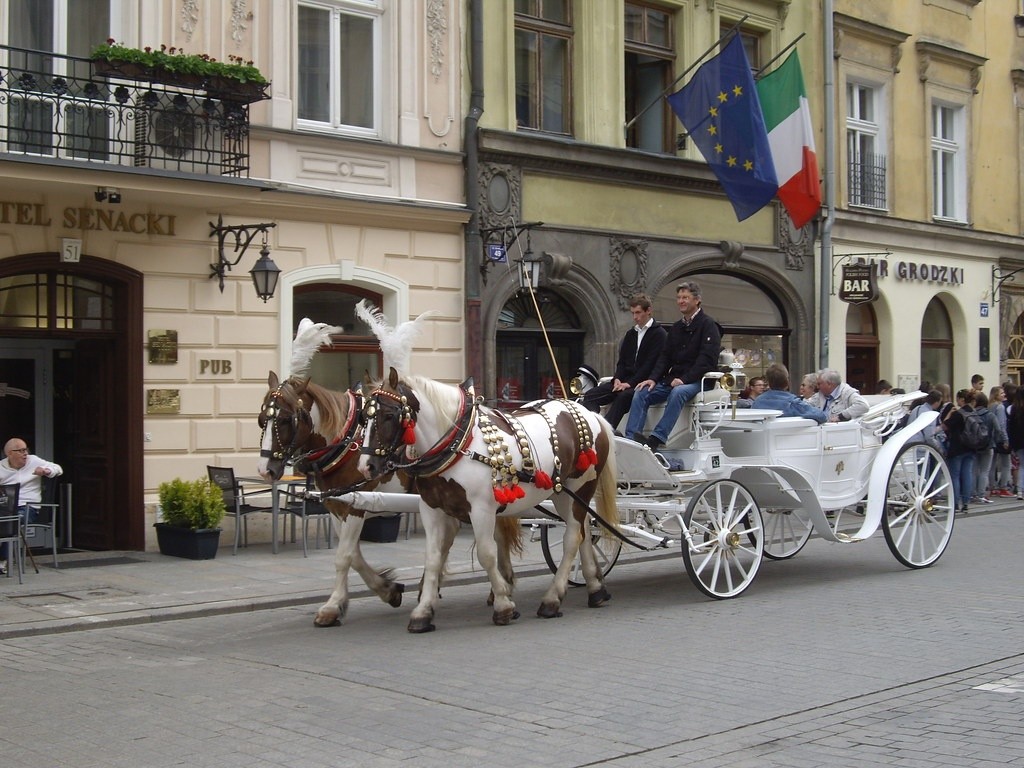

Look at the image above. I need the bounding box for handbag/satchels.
[935,431,948,450]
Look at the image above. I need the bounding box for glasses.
[752,384,767,387]
[675,292,696,301]
[11,448,30,453]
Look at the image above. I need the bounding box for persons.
[614,281,721,453]
[0,439,63,573]
[877,374,1024,514]
[817,369,869,422]
[750,362,826,424]
[800,373,821,408]
[737,377,764,409]
[582,297,667,428]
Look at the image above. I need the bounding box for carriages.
[257,298,955,636]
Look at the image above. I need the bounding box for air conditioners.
[118,91,222,177]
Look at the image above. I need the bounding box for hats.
[575,364,600,387]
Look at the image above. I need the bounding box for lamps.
[479,222,549,305]
[95,187,121,204]
[208,214,283,302]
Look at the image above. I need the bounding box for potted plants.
[153,475,226,560]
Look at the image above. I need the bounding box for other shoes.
[1000,490,1017,498]
[961,507,969,513]
[1016,491,1023,500]
[614,429,626,437]
[633,431,654,450]
[955,505,960,513]
[976,495,994,504]
[991,489,1001,497]
[826,510,835,519]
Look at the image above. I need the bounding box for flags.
[666,32,778,223]
[757,47,821,230]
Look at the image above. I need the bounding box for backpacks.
[957,404,991,450]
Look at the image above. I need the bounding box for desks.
[236,476,308,556]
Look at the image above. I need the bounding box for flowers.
[90,36,266,83]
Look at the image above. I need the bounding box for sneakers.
[0,559,7,574]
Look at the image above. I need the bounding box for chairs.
[0,476,58,585]
[206,465,262,557]
[276,474,332,557]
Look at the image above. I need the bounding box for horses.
[257,366,622,632]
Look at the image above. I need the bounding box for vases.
[92,59,263,102]
